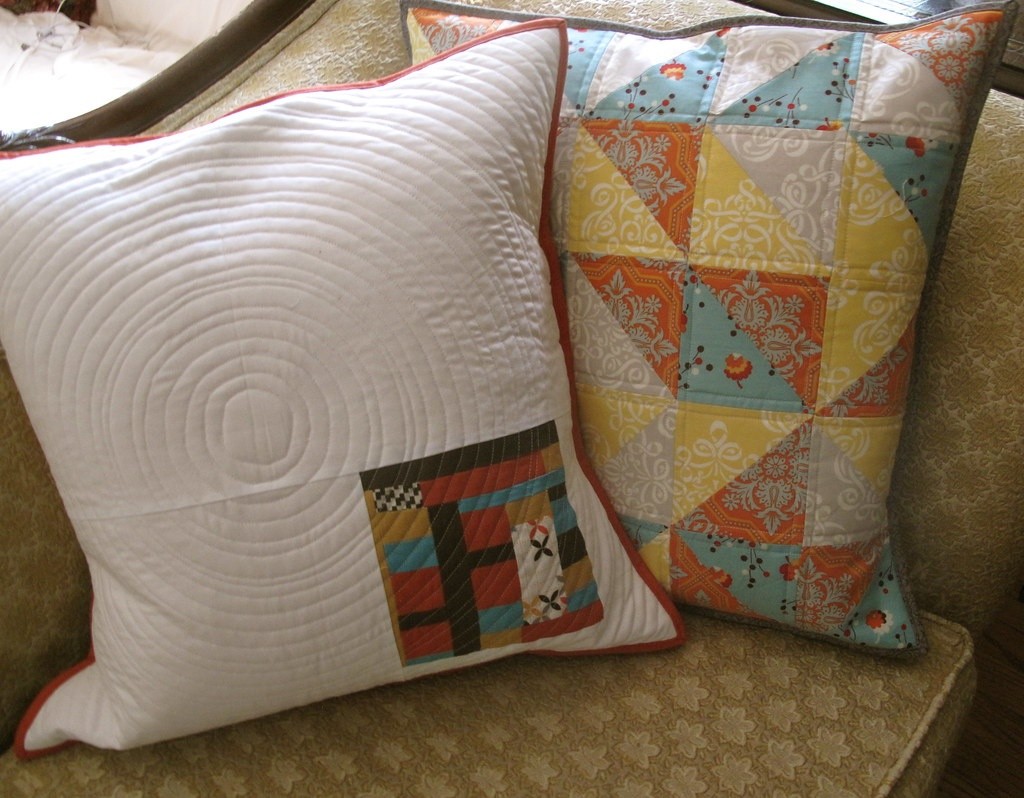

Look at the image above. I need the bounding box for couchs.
[0,0,1024,798]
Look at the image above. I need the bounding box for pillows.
[0,17,687,762]
[399,0,1018,662]
